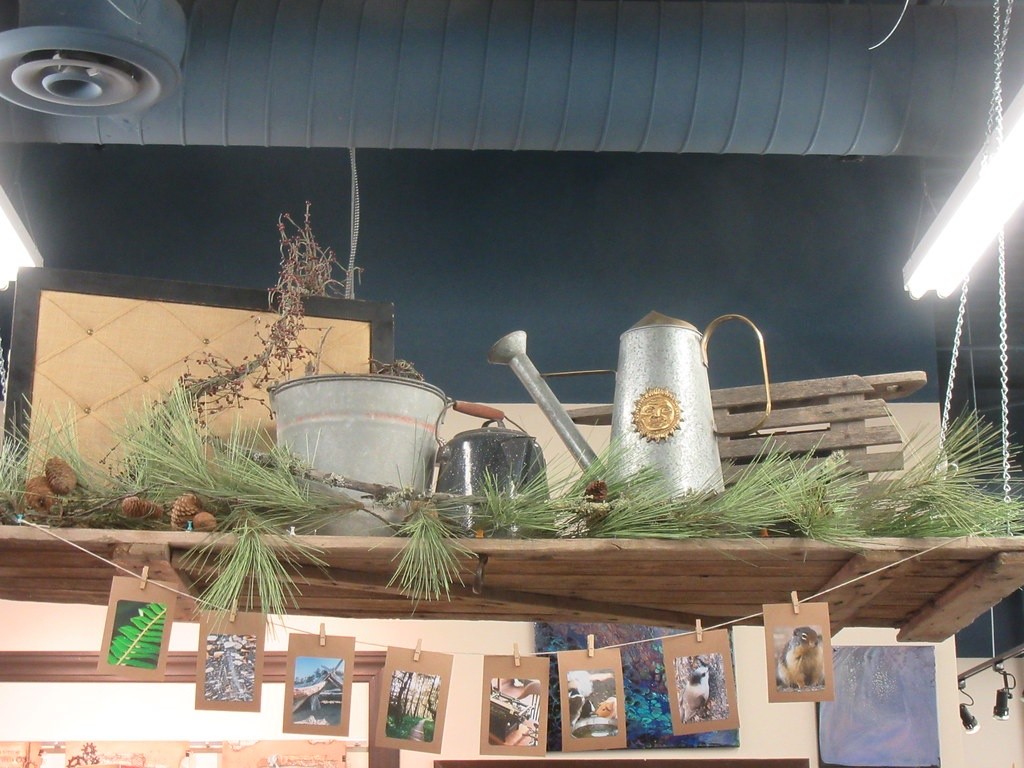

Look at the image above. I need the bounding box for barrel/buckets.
[265,371,453,537]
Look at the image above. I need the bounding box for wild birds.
[684,667,710,721]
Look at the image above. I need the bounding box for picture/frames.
[0,647,406,768]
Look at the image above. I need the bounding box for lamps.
[993,664,1016,722]
[902,85,1024,300]
[957,680,981,735]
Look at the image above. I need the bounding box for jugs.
[431,400,557,539]
[488,308,773,507]
